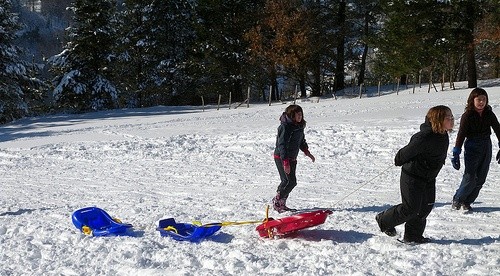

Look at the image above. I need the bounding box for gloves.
[496,150,500,164]
[451,147,462,170]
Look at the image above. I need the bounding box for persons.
[272,105,315,214]
[449,88,500,210]
[375,104,454,244]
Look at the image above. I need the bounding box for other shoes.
[462,202,472,211]
[272,193,282,213]
[376,214,397,237]
[452,195,462,210]
[403,235,430,244]
[281,198,294,212]
